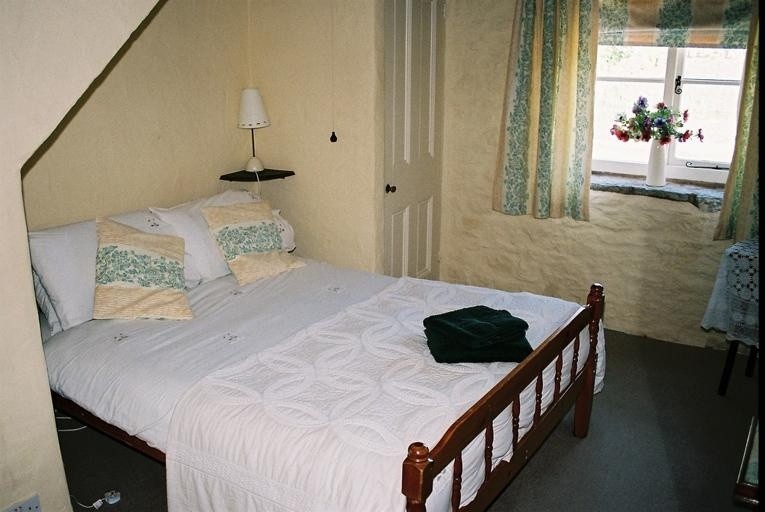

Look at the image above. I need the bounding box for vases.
[646,139,669,187]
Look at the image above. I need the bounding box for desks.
[219,169,295,200]
[699,237,759,396]
[732,416,761,509]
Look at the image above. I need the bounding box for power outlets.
[8,494,40,512]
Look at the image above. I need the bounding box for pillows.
[32,265,63,335]
[91,214,196,322]
[149,189,296,286]
[28,210,204,331]
[200,202,297,287]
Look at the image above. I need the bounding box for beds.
[37,253,607,512]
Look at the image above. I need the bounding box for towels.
[423,305,534,364]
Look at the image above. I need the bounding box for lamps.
[236,87,270,173]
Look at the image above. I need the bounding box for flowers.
[610,97,705,148]
